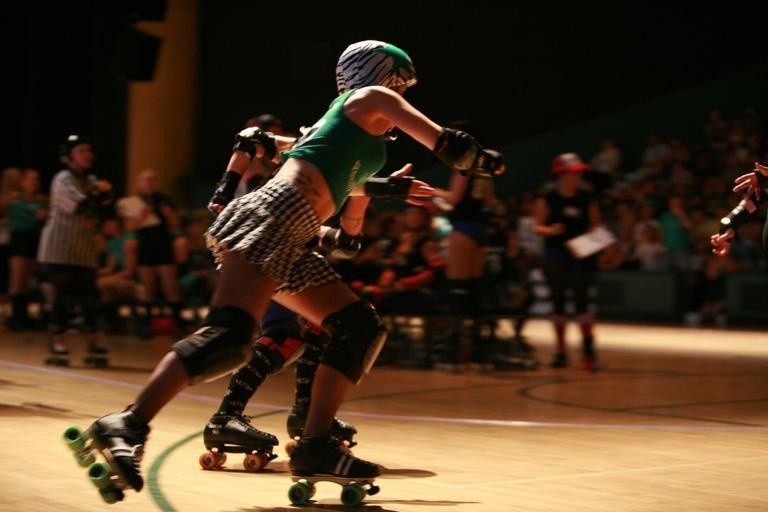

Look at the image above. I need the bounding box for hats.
[547,152,590,175]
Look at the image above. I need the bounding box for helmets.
[336,40,418,95]
[58,132,93,157]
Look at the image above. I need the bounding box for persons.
[337,104,768,370]
[63,40,508,504]
[200,115,433,472]
[1,116,360,475]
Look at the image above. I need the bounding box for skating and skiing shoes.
[64,409,150,504]
[43,344,68,367]
[86,344,109,370]
[285,408,382,507]
[199,412,280,472]
